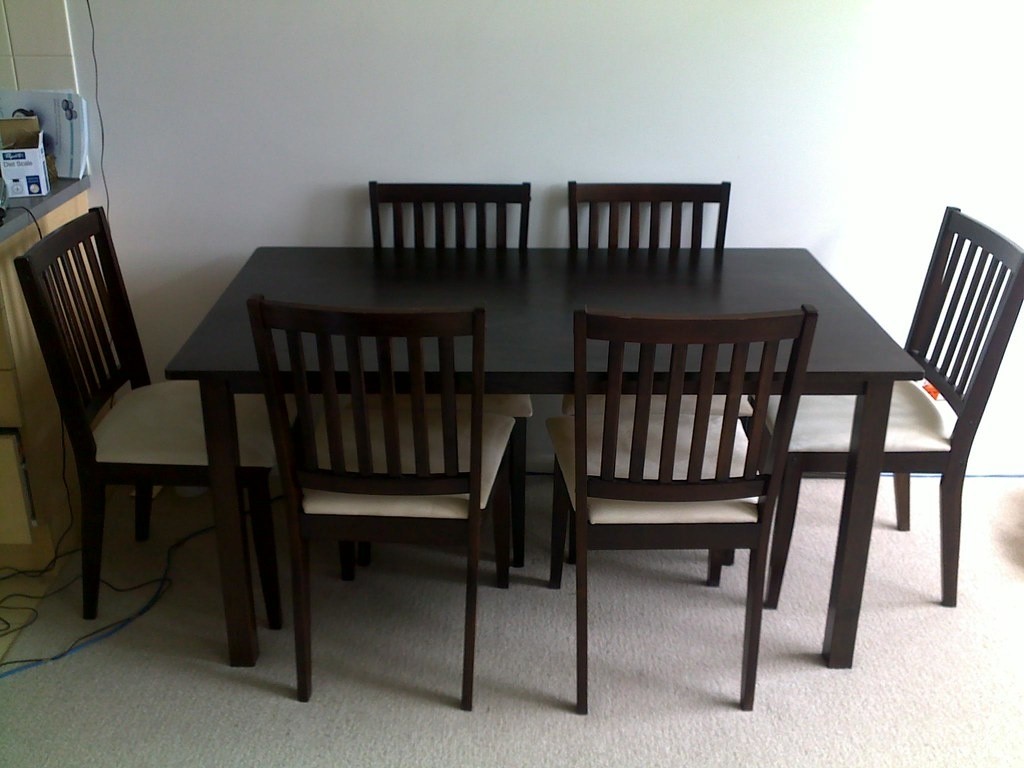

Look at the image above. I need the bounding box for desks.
[166,246,926,670]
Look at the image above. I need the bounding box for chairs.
[15,206,301,628]
[740,206,1024,607]
[247,181,532,710]
[552,180,818,717]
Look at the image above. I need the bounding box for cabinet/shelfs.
[0,191,120,574]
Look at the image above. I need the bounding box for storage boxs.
[0,117,50,198]
